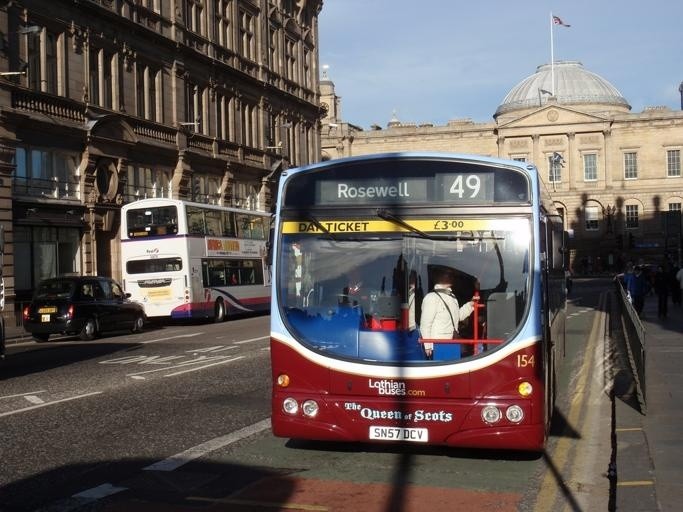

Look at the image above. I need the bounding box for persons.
[616,248,683,321]
[421,266,480,357]
[318,260,373,311]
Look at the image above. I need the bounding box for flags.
[552,15,571,27]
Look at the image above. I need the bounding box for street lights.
[599,203,616,237]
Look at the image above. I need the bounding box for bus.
[268,151,570,458]
[116,200,275,322]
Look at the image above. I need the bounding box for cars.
[608,263,661,289]
[24,276,146,341]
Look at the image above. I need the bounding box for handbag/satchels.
[453,328,462,340]
